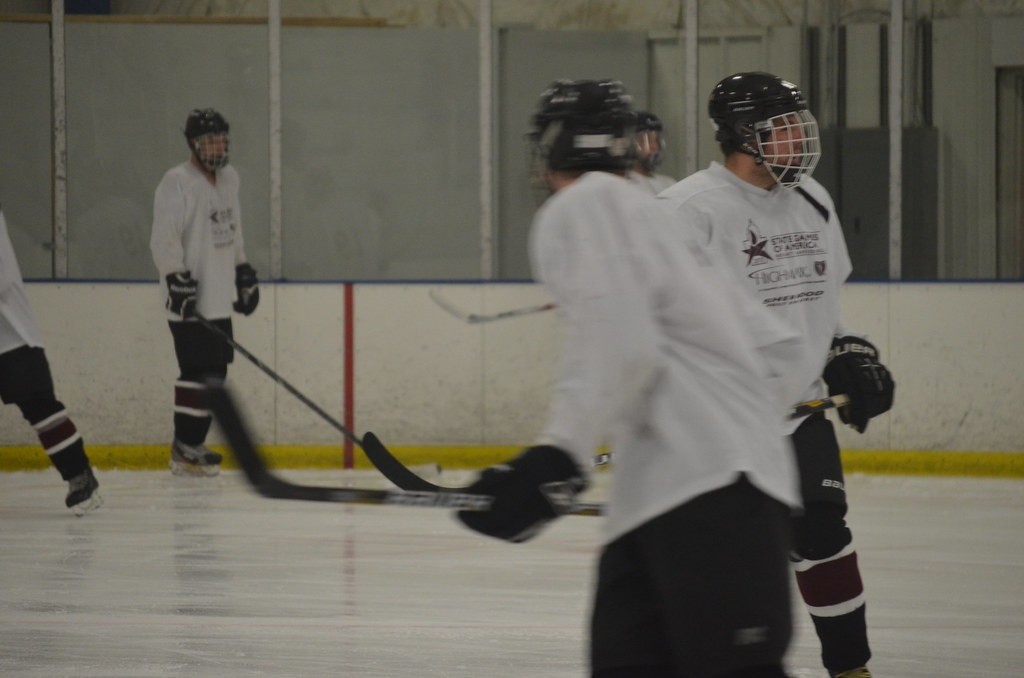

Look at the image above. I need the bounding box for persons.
[453,72,896,678]
[148,108,261,477]
[0,214,105,519]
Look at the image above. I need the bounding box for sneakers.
[64,464,101,517]
[167,439,220,478]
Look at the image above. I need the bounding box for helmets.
[708,70,821,189]
[630,110,667,175]
[182,107,231,170]
[521,76,635,175]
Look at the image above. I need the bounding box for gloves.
[455,444,588,543]
[165,270,198,320]
[821,336,896,435]
[231,263,259,315]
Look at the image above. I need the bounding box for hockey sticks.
[362,392,849,496]
[201,379,607,520]
[191,310,362,448]
[426,289,557,325]
